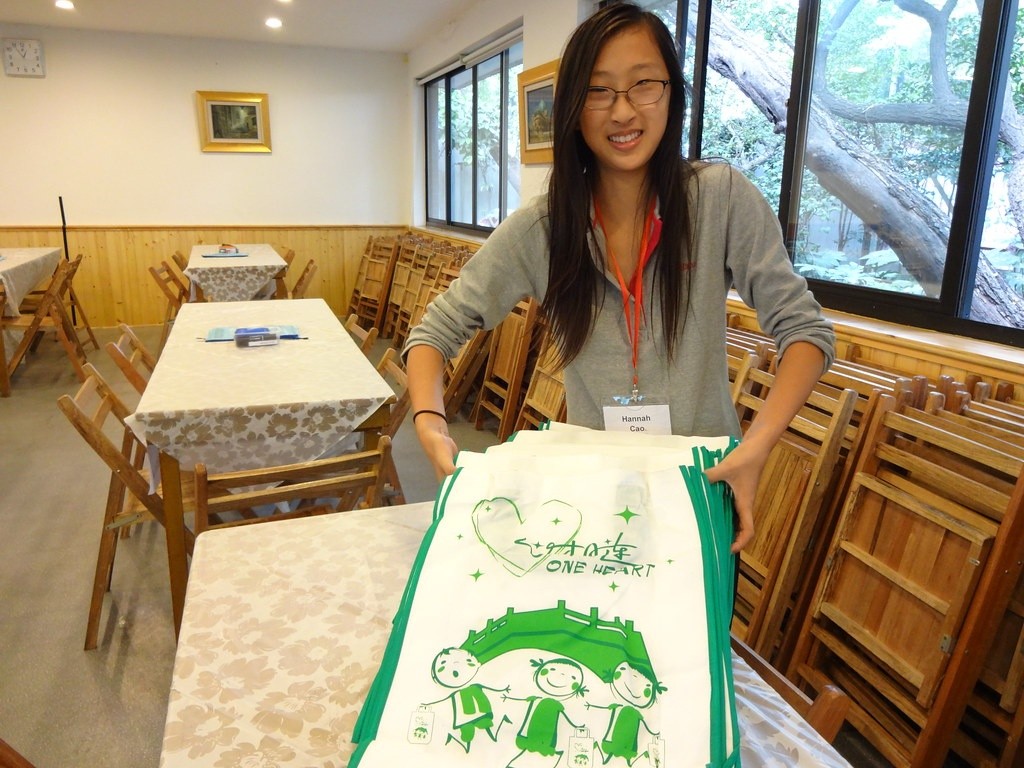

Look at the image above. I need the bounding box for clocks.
[1,38,46,77]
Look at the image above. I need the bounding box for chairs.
[2,229,1024,767]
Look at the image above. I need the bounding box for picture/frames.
[517,57,560,164]
[195,90,272,153]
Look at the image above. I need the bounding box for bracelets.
[413,409,448,426]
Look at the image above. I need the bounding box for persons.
[400,5,837,635]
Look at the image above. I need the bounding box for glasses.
[581,78,673,112]
